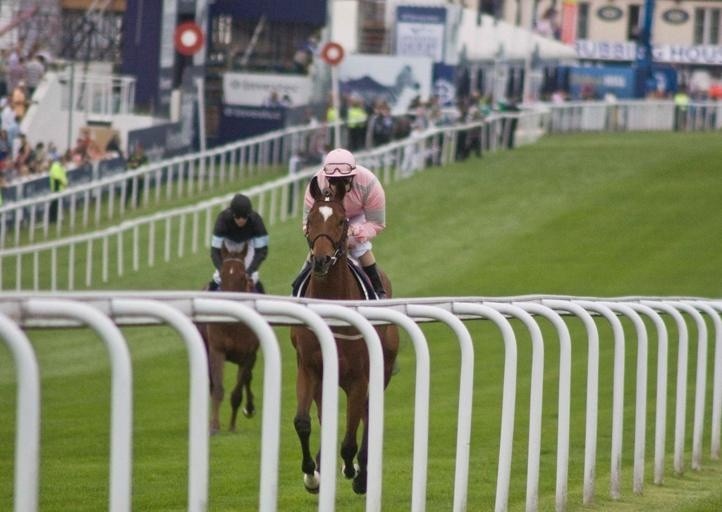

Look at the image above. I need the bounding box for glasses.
[233,214,249,219]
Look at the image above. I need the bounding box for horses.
[192,239,266,434]
[290,175,400,495]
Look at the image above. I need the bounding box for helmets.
[323,148,358,193]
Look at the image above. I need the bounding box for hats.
[227,192,253,215]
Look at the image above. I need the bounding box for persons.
[539,76,602,133]
[291,146,386,301]
[200,192,270,293]
[643,75,722,132]
[407,79,521,167]
[120,139,151,208]
[50,152,69,224]
[1,34,129,205]
[266,93,399,169]
